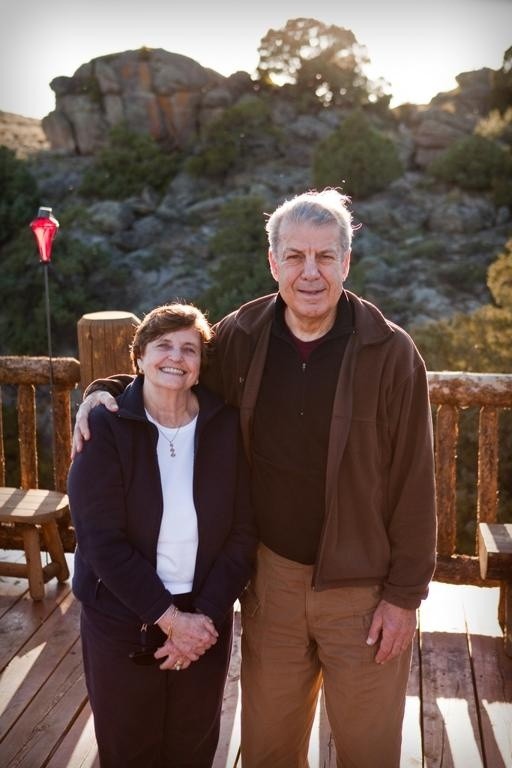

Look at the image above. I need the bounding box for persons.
[71,186,437,768]
[66,303,260,767]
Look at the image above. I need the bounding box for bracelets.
[167,607,178,639]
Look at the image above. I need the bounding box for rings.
[175,660,183,670]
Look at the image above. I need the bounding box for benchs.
[1,484,71,606]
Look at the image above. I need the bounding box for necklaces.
[151,414,187,458]
